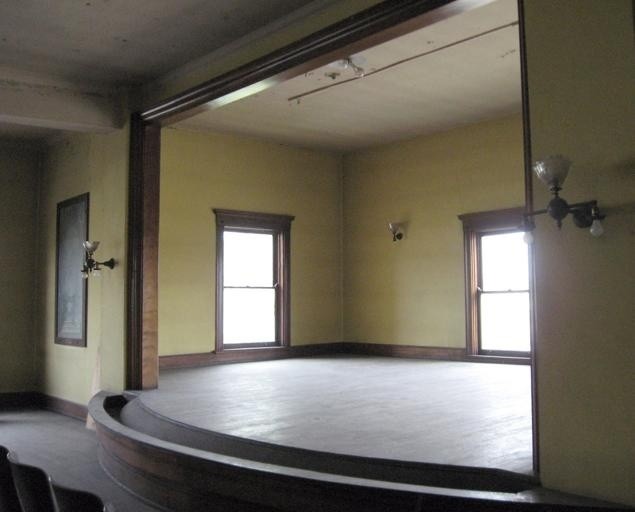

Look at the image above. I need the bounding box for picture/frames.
[55,192,91,348]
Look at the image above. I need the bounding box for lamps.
[518,154,605,245]
[79,240,116,279]
[387,221,403,241]
